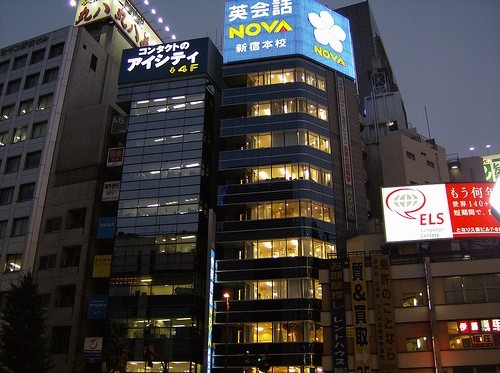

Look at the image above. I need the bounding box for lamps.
[135,1,176,41]
